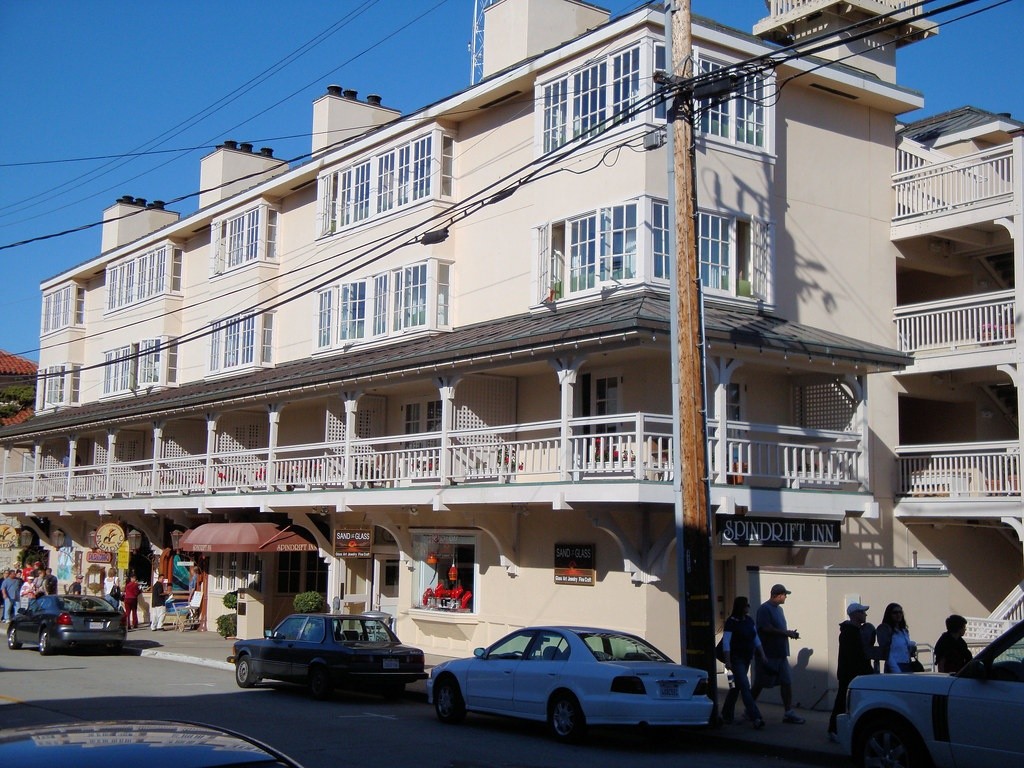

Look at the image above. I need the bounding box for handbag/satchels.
[715,637,735,670]
[911,656,924,672]
[110,577,120,602]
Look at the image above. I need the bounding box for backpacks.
[68,584,74,595]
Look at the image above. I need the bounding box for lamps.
[88,528,98,553]
[427,545,437,563]
[22,530,33,550]
[448,545,458,580]
[170,528,183,555]
[128,529,141,554]
[51,529,65,551]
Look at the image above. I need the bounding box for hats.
[34,562,41,567]
[77,574,84,578]
[771,585,790,595]
[847,604,869,615]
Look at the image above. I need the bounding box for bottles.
[727,669,735,688]
[910,654,916,662]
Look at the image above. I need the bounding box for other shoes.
[831,732,840,742]
[753,718,765,728]
[723,716,732,723]
[127,627,131,629]
[6,620,10,623]
[133,625,138,628]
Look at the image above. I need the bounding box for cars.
[225,610,429,699]
[6,594,128,656]
[1,719,304,768]
[826,618,1024,768]
[425,625,716,742]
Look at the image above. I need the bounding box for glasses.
[747,604,752,608]
[893,610,904,614]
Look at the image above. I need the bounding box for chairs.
[343,631,359,641]
[543,646,561,658]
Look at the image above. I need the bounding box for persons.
[72,575,84,595]
[719,596,768,731]
[876,602,917,673]
[741,584,806,726]
[103,568,120,610]
[62,450,81,499]
[934,615,972,673]
[827,603,878,742]
[0,560,57,624]
[124,574,142,629]
[150,574,169,631]
[165,583,173,600]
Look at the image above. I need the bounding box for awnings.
[177,522,319,552]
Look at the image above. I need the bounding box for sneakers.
[782,711,805,724]
[742,708,751,720]
